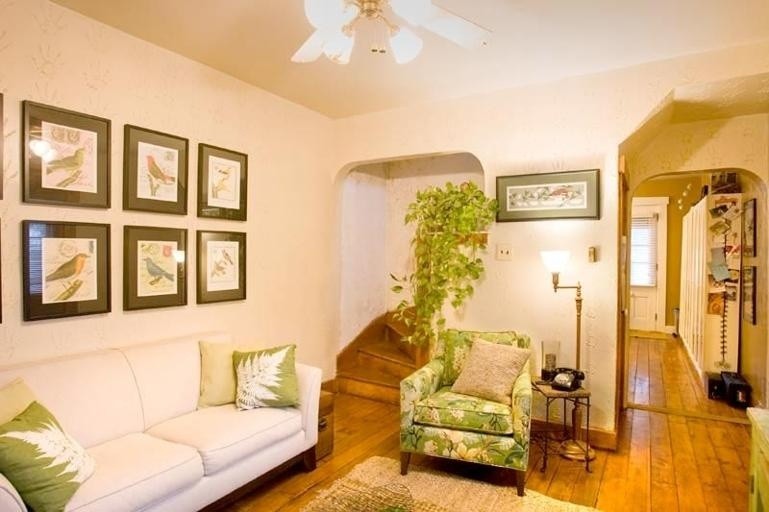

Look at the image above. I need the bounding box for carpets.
[297,455,602,512]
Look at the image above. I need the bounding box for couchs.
[0,332,322,512]
[398,330,533,499]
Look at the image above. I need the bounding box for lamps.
[303,0,433,66]
[540,250,596,463]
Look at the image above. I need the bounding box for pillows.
[196,339,271,408]
[0,377,39,426]
[453,340,533,407]
[0,402,98,512]
[233,343,303,413]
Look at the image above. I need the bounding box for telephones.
[550,367,585,392]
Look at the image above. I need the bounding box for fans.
[290,0,492,69]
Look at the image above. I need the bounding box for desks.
[533,375,593,475]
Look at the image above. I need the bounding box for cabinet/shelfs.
[680,194,740,384]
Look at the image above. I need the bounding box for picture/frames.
[197,143,248,222]
[122,123,188,214]
[741,198,757,259]
[495,168,602,223]
[19,99,112,208]
[21,219,112,321]
[122,225,188,311]
[742,264,758,327]
[196,230,247,305]
